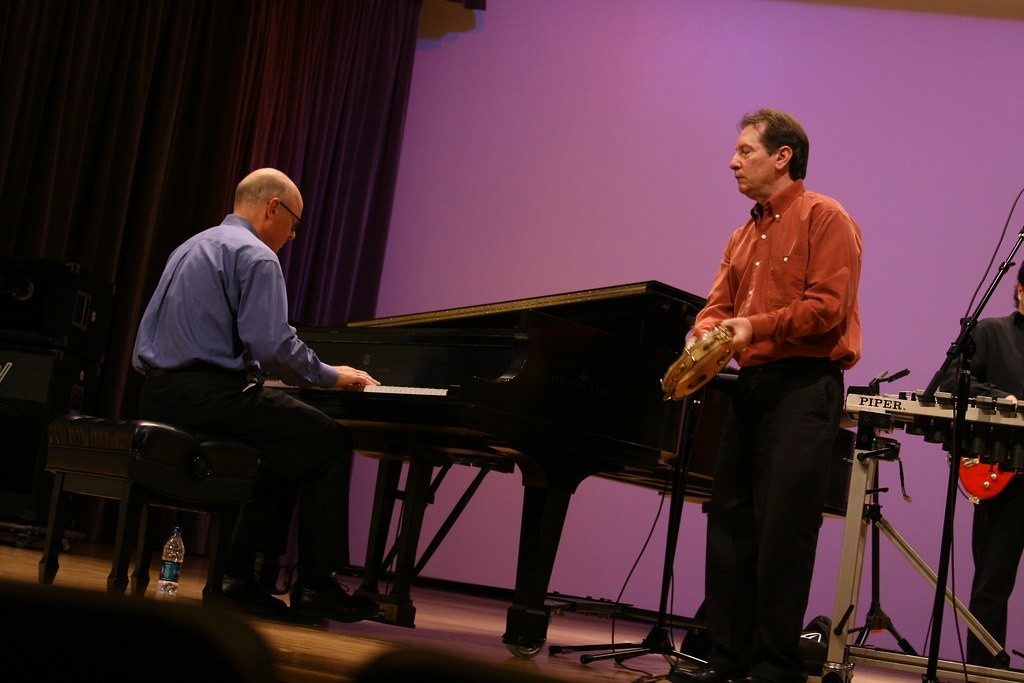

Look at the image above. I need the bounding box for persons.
[939,259,1024,671]
[132,167,382,630]
[669,105,863,683]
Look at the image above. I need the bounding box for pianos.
[258,280,857,658]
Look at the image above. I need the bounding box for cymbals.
[661,324,736,402]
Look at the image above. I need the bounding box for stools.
[39,416,284,597]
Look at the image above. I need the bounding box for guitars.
[959,454,1019,501]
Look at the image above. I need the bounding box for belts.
[144,360,219,381]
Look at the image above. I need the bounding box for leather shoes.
[289,575,379,623]
[669,665,730,683]
[203,577,288,619]
[726,674,767,683]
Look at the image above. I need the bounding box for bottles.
[157,525,186,593]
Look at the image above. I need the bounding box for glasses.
[268,199,302,231]
